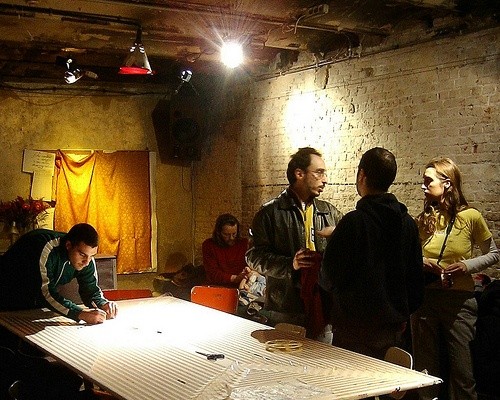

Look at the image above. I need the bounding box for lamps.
[114,25,152,75]
[64,56,84,84]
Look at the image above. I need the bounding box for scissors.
[195,351,224,360]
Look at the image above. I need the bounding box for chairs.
[383,346,413,399]
[190,286,240,312]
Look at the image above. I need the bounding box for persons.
[413,158,500,400]
[202,147,423,361]
[0,223,119,358]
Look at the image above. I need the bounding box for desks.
[0,296,444,400]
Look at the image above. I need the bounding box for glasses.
[294,166,327,180]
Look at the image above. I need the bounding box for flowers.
[0,195,52,227]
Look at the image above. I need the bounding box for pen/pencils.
[91,300,97,309]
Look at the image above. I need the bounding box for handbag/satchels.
[439,270,474,295]
